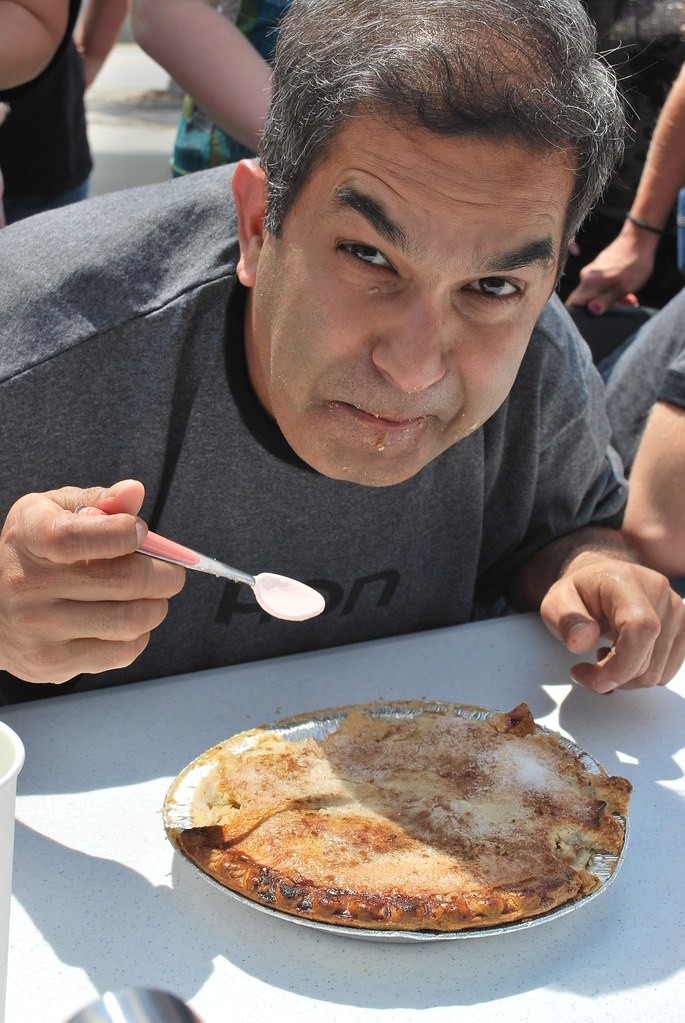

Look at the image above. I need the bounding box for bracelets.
[626,213,664,236]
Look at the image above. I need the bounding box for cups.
[1,722,26,1021]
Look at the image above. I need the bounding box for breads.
[178,703,633,932]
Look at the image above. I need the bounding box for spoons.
[73,505,325,622]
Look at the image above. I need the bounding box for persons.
[0,0,685,695]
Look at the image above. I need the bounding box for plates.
[163,700,630,943]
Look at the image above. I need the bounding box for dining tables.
[2,594,685,1023]
